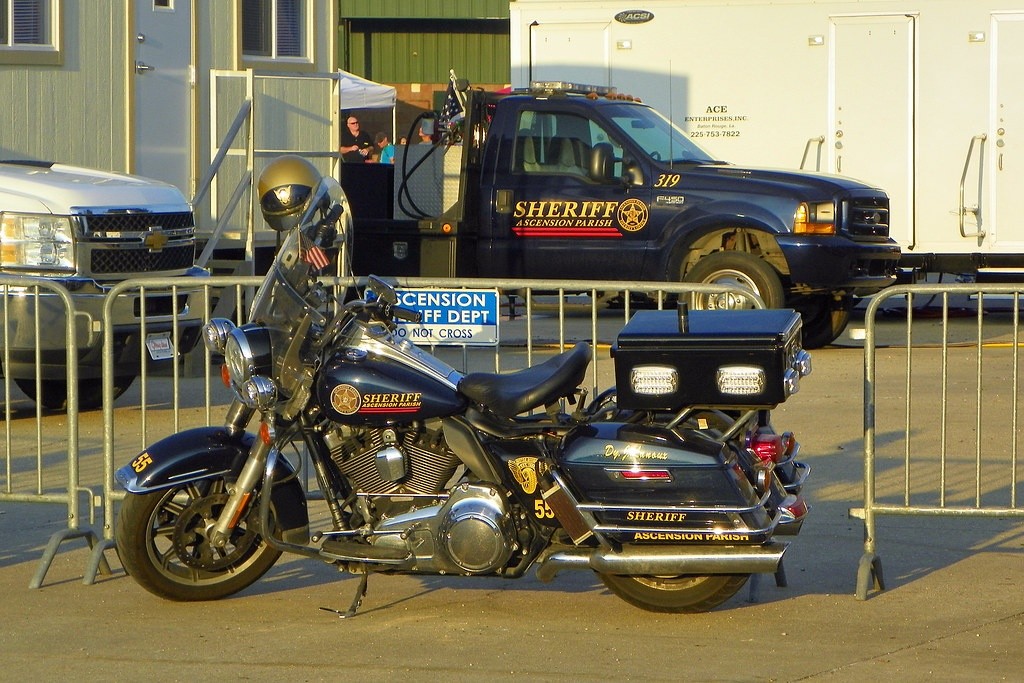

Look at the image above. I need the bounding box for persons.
[372,132,397,164]
[340,116,375,164]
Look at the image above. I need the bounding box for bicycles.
[111,158,813,619]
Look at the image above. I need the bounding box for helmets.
[257,155,331,230]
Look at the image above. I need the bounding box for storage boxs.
[610,309,812,410]
[561,423,775,545]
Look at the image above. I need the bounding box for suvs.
[0,145,210,415]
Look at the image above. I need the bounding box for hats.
[374,132,388,144]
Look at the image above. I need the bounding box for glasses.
[351,122,359,125]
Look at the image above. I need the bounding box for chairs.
[515,128,583,175]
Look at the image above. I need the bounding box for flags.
[438,76,466,124]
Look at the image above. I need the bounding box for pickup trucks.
[205,67,901,350]
